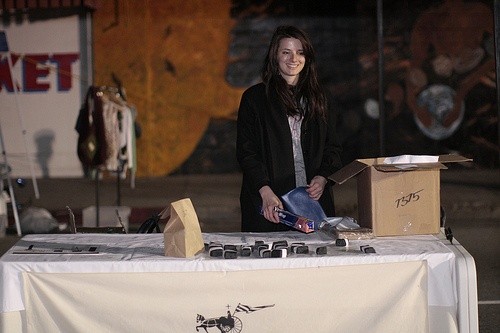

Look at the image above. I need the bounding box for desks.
[1,226,479,332]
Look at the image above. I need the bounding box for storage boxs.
[328,153,472,237]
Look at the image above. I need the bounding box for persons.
[235,23,347,232]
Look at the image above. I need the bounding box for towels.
[74,85,143,188]
